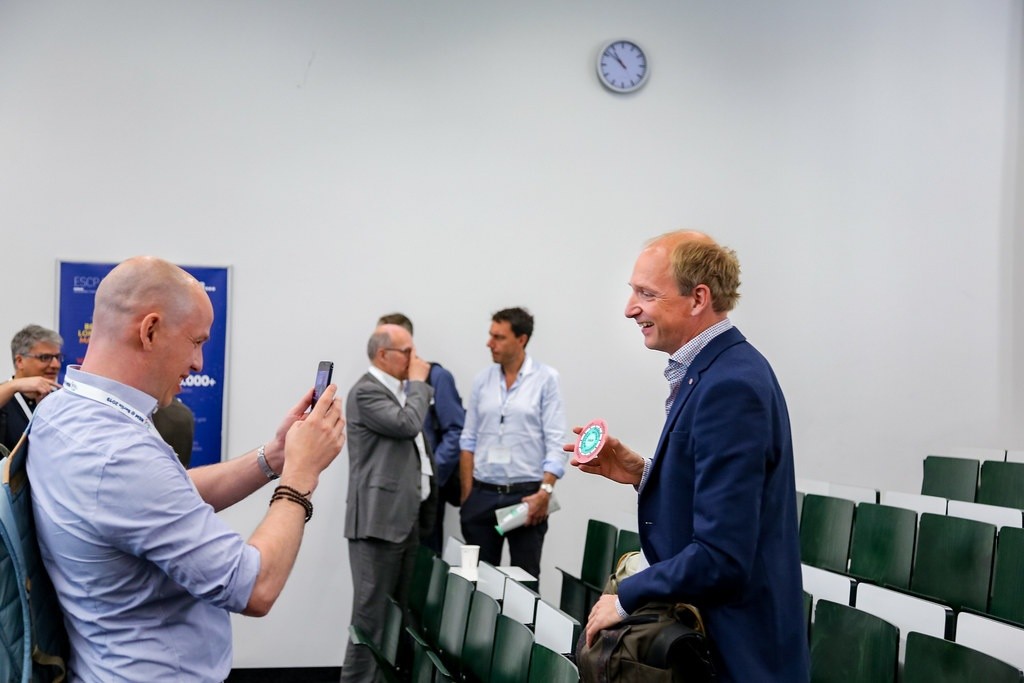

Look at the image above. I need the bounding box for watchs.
[270,486,313,523]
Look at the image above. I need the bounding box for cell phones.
[311,361,334,412]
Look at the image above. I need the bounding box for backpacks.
[0,396,69,682]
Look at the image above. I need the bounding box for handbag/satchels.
[575,599,716,683]
[426,362,461,508]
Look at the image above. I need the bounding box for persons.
[27,256,346,683]
[456,307,568,595]
[154,397,194,467]
[0,326,65,457]
[339,324,435,683]
[564,231,811,683]
[376,314,467,555]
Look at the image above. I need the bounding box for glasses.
[18,353,65,364]
[385,347,411,361]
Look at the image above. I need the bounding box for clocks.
[597,40,650,93]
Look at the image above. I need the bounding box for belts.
[473,478,542,497]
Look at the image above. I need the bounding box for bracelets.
[257,446,279,480]
[540,484,552,493]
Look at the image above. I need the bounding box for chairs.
[347,456,1024,683]
